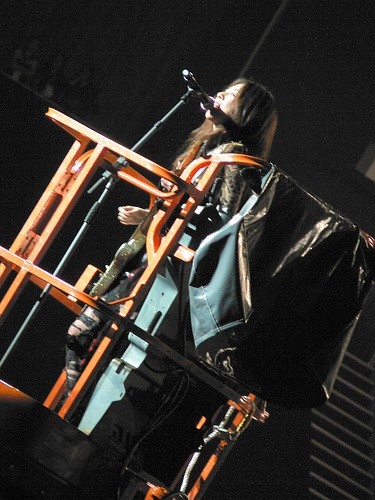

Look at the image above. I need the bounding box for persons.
[63,77,278,430]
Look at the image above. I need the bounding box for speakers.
[0,382,121,500]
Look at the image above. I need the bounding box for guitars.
[88,138,211,297]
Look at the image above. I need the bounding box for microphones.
[183,69,215,110]
